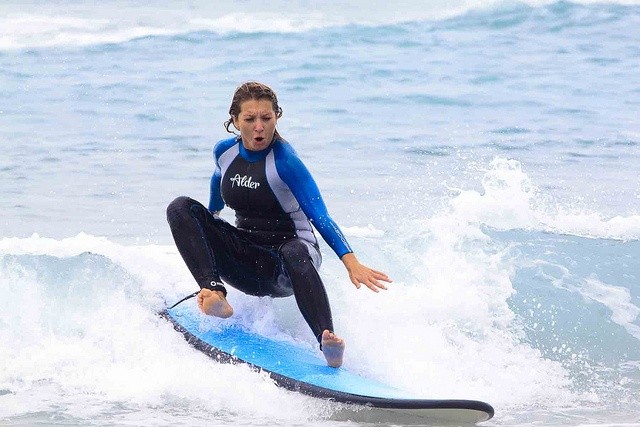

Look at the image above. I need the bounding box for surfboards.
[161,290,495,426]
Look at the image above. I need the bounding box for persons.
[166,82,392,368]
